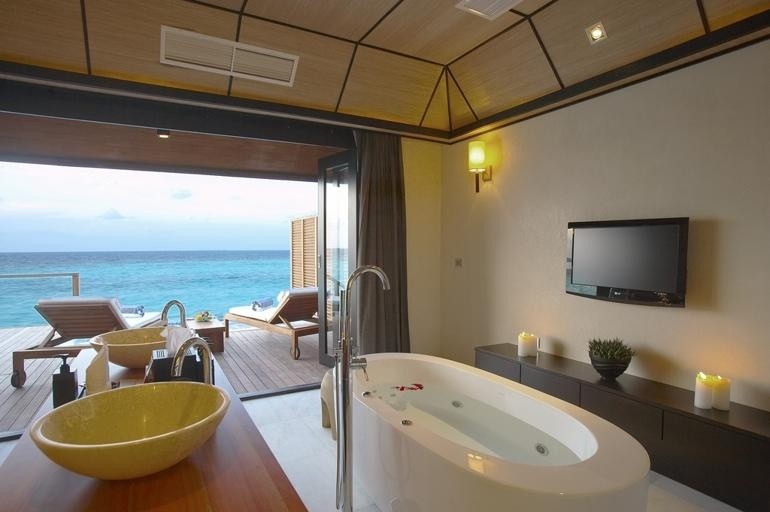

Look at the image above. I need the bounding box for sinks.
[93,327,172,370]
[31,381,229,481]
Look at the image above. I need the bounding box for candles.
[530,333,538,356]
[713,376,730,411]
[518,332,529,357]
[695,372,712,409]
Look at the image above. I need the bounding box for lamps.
[468,142,486,193]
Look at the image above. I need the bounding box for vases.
[587,336,635,378]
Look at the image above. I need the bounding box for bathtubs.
[342,350,650,512]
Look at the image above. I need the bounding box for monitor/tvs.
[565,216,690,307]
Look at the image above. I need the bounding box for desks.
[185,318,229,353]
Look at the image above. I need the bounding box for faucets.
[334,263,392,512]
[170,338,213,387]
[161,300,187,330]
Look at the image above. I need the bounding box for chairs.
[225,289,319,359]
[11,299,167,389]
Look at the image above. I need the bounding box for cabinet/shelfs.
[476,343,769,512]
